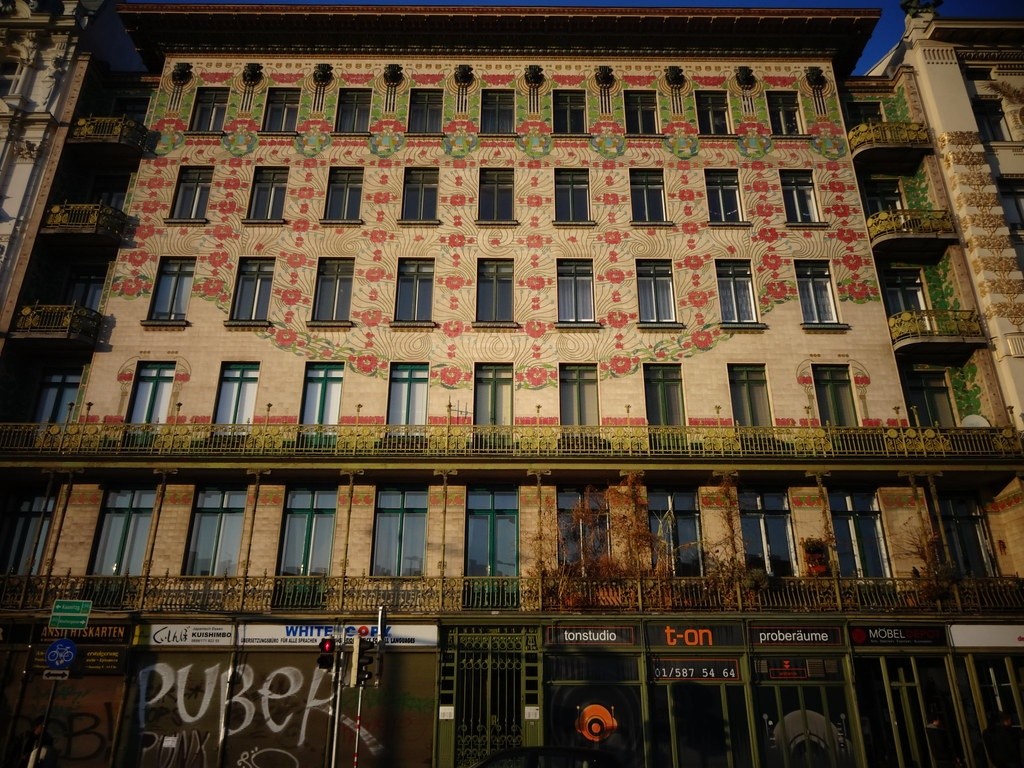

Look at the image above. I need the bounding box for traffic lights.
[350,635,372,688]
[317,637,335,669]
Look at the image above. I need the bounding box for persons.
[926,711,963,768]
[983,710,1024,768]
[19,715,55,768]
